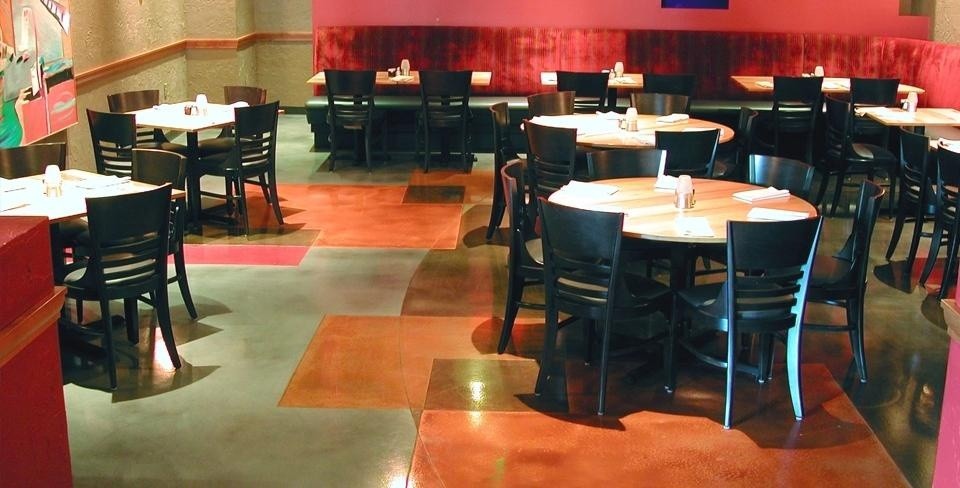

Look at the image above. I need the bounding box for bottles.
[609,61,624,79]
[396,59,410,76]
[677,175,694,209]
[617,106,639,131]
[45,163,61,200]
[184,94,207,115]
[907,91,919,112]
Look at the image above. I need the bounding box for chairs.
[497,161,595,364]
[534,197,675,416]
[485,70,960,300]
[769,178,884,383]
[198,100,284,235]
[198,86,270,209]
[417,69,473,173]
[107,89,188,159]
[0,142,89,257]
[54,182,182,390]
[323,69,377,174]
[675,215,825,429]
[72,149,197,324]
[86,108,136,179]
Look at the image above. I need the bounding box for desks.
[307,72,491,162]
[548,177,817,378]
[123,103,284,235]
[0,169,186,344]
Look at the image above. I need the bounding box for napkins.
[0,193,30,211]
[75,175,131,188]
[0,178,27,190]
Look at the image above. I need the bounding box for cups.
[815,65,825,77]
[388,67,396,77]
